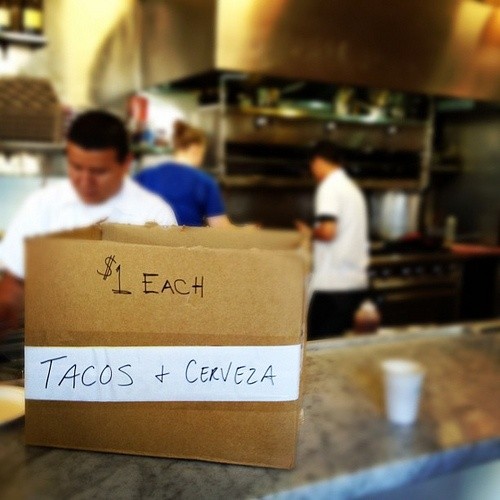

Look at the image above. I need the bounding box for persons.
[136,118,260,229]
[0,110,179,327]
[295,139,372,342]
[428,160,500,322]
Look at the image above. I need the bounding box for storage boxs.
[24,221,313,470]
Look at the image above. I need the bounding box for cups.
[382,357,425,426]
